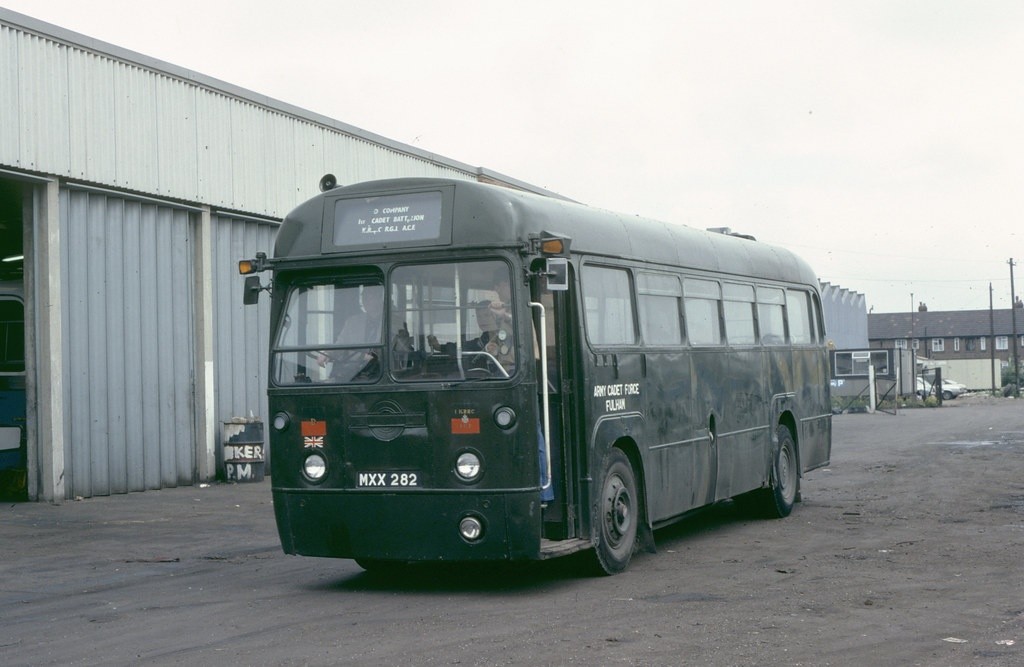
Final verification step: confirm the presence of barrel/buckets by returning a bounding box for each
[223,421,266,483]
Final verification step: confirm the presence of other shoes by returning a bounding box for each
[541,501,548,508]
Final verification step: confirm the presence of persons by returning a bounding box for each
[317,286,382,381]
[427,301,496,369]
[485,264,555,501]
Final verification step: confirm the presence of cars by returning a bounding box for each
[916,378,968,400]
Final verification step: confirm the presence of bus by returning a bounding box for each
[0,279,25,499]
[239,176,832,577]
